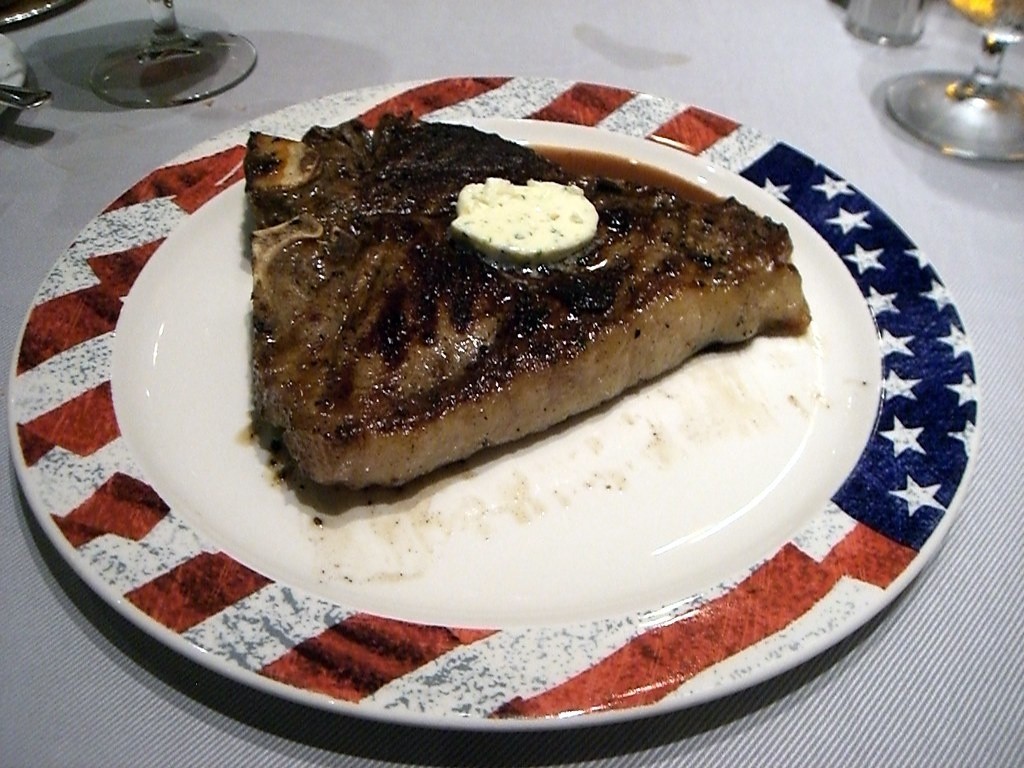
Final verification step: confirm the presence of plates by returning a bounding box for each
[0,33,28,117]
[6,78,984,731]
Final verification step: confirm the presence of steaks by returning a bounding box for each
[239,110,812,489]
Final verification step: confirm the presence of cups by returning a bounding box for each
[843,0,928,46]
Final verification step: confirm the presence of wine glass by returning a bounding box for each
[87,1,258,109]
[886,0,1023,161]
